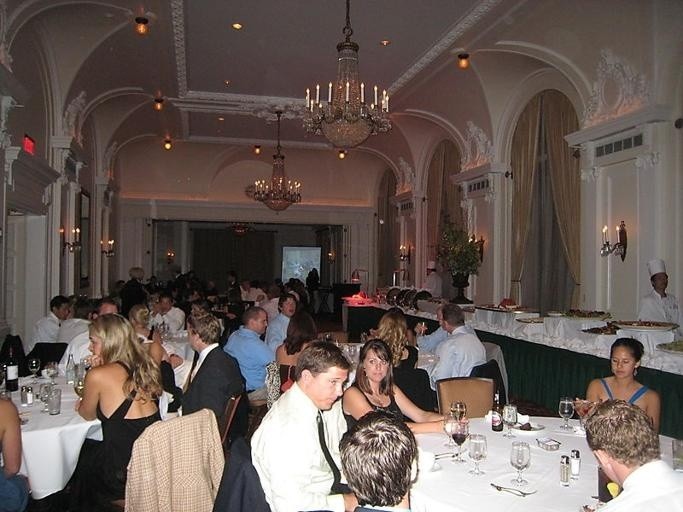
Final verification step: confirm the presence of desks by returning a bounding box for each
[347,301,683,441]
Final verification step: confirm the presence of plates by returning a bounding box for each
[464,302,540,314]
[561,313,612,321]
[610,320,679,331]
[657,342,683,356]
[386,287,433,311]
[547,310,564,317]
[581,328,618,336]
[513,318,543,324]
[516,422,546,432]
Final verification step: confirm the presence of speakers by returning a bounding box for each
[333,284,360,321]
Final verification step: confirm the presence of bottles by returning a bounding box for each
[5,343,19,392]
[65,353,76,386]
[560,450,581,487]
[21,385,33,407]
[491,388,504,431]
[78,359,90,379]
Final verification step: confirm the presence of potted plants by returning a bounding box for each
[433,222,483,304]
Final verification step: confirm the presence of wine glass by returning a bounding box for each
[510,439,531,487]
[558,396,575,432]
[501,404,517,441]
[47,361,59,386]
[38,382,53,414]
[322,332,358,366]
[468,434,488,476]
[443,400,469,464]
[27,358,41,386]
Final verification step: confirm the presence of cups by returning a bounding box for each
[156,326,192,343]
[670,439,683,472]
[48,389,61,415]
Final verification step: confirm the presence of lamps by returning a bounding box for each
[350,268,369,282]
[304,0,393,160]
[458,52,470,69]
[60,223,83,257]
[135,17,172,151]
[255,145,261,154]
[399,244,411,264]
[99,237,115,257]
[600,219,627,264]
[226,221,257,237]
[251,110,302,218]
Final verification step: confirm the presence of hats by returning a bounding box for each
[427,261,435,269]
[648,259,666,277]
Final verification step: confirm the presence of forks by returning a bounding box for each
[492,484,540,503]
[435,451,452,460]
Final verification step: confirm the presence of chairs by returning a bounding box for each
[121,357,280,512]
[387,339,511,420]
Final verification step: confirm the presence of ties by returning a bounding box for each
[317,417,341,489]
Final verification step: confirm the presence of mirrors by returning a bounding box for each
[80,185,91,288]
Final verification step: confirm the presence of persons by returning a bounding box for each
[35,296,246,495]
[579,397,683,510]
[377,302,487,407]
[116,265,316,406]
[250,339,468,511]
[0,397,30,511]
[574,337,661,433]
[637,273,679,323]
